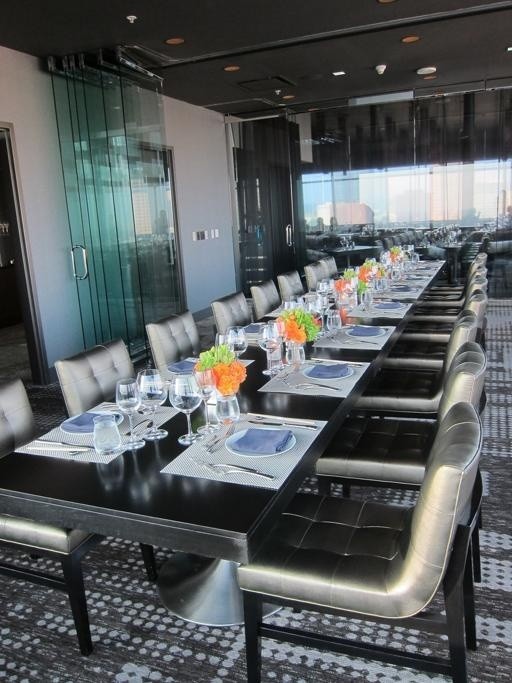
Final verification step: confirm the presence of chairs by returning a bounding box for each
[415,230,423,240]
[461,232,489,276]
[392,236,402,246]
[350,309,482,418]
[236,402,483,683]
[211,291,252,334]
[250,279,281,319]
[145,310,201,369]
[277,270,304,302]
[315,341,487,583]
[428,264,488,294]
[400,233,409,244]
[304,262,328,290]
[399,274,488,349]
[374,239,385,260]
[54,336,137,418]
[383,237,393,250]
[418,254,486,310]
[406,231,416,242]
[389,289,488,366]
[411,267,488,326]
[0,375,157,656]
[317,256,337,277]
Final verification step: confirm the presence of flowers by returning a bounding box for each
[390,246,401,262]
[276,306,321,344]
[194,345,247,395]
[357,261,386,283]
[335,268,367,295]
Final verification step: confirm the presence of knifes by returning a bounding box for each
[248,420,319,430]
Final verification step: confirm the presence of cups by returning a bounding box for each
[214,331,234,353]
[215,392,241,426]
[360,286,372,312]
[93,414,122,454]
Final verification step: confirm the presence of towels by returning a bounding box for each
[170,360,198,373]
[309,364,349,379]
[392,286,411,292]
[351,326,380,337]
[377,302,400,309]
[244,324,262,332]
[62,411,119,433]
[407,276,422,280]
[418,266,431,270]
[232,428,292,453]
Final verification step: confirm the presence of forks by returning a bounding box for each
[206,425,236,453]
[191,457,259,473]
[203,463,276,480]
[280,379,339,391]
[291,386,341,390]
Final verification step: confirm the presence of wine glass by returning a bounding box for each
[194,370,220,434]
[286,342,304,372]
[135,369,168,442]
[327,310,341,342]
[169,372,204,445]
[225,326,248,359]
[268,320,288,369]
[116,378,146,450]
[258,324,282,376]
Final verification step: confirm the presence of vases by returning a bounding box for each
[215,396,240,424]
[286,341,305,364]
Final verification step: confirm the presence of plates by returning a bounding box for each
[303,366,354,381]
[60,410,125,436]
[225,427,297,458]
[373,304,406,312]
[345,329,386,338]
